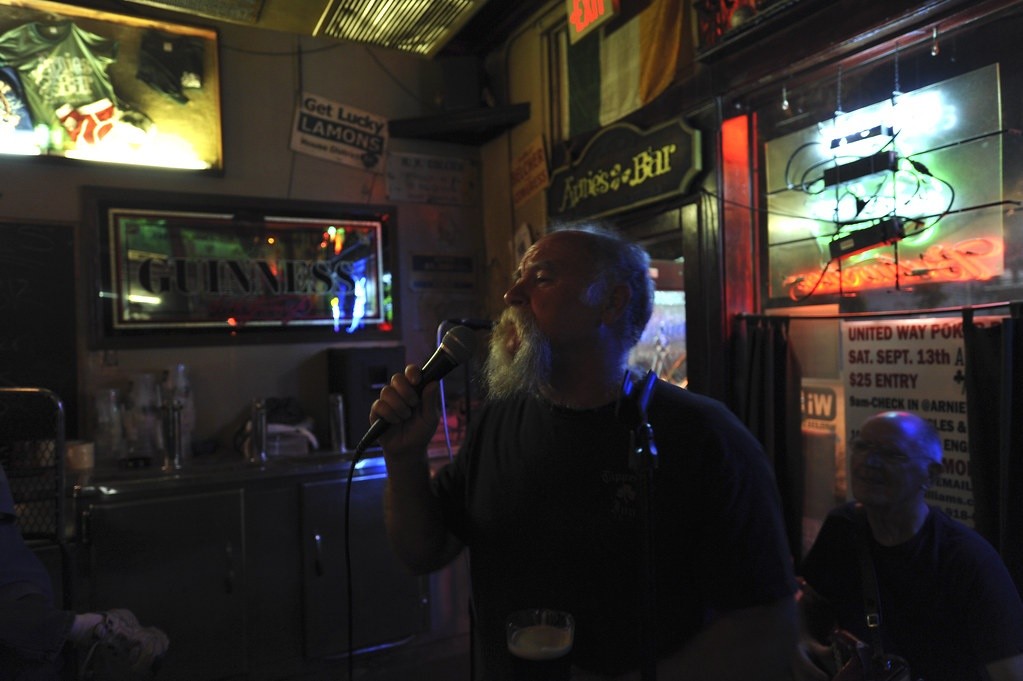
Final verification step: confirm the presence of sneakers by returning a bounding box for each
[83,607,169,677]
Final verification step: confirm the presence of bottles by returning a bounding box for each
[96,362,195,462]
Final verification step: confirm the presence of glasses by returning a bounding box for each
[848,437,939,467]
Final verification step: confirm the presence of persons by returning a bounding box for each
[791,410,1023,681]
[371,229,797,681]
[0,466,170,681]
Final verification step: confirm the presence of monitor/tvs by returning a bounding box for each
[759,61,1009,311]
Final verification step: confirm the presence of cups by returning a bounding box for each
[504,609,575,681]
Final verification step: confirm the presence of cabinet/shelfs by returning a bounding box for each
[81,450,482,681]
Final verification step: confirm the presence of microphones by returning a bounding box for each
[351,324,479,460]
